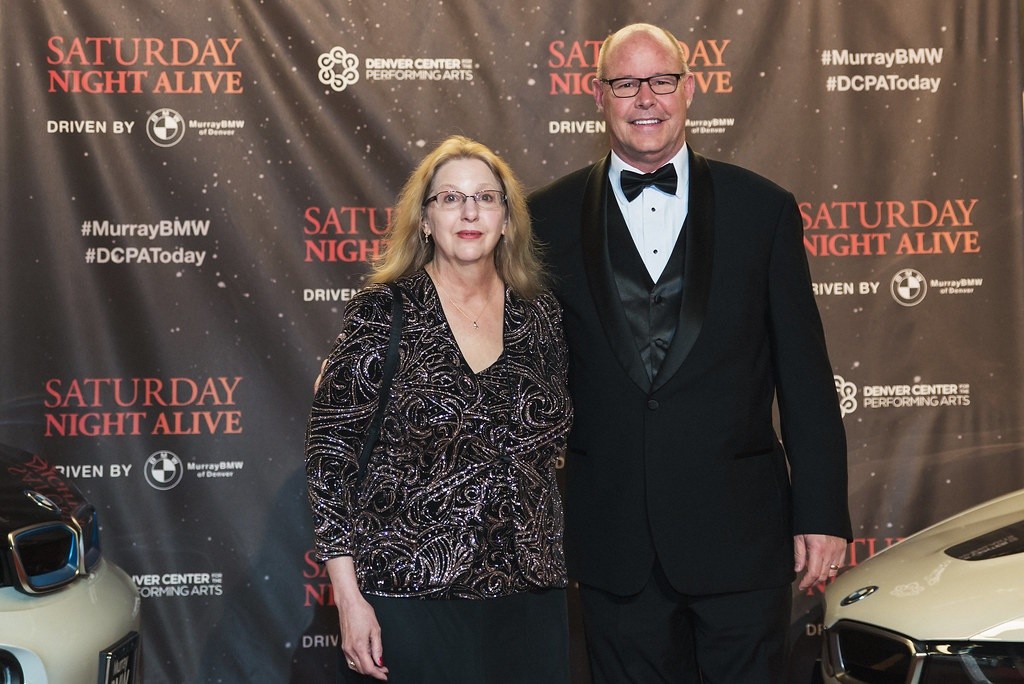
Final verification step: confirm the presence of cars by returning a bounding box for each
[789,486,1024,684]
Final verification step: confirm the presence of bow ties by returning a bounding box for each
[620,163,679,202]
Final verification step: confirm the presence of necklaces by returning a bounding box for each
[424,265,499,331]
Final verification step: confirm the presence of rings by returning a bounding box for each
[830,564,838,570]
[350,661,356,668]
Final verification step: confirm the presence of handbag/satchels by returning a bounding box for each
[289,604,358,684]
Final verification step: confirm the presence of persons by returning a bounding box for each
[524,23,854,684]
[304,136,576,683]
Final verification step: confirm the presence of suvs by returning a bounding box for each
[0,443,142,684]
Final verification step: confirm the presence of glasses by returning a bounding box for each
[422,190,508,209]
[598,70,687,98]
